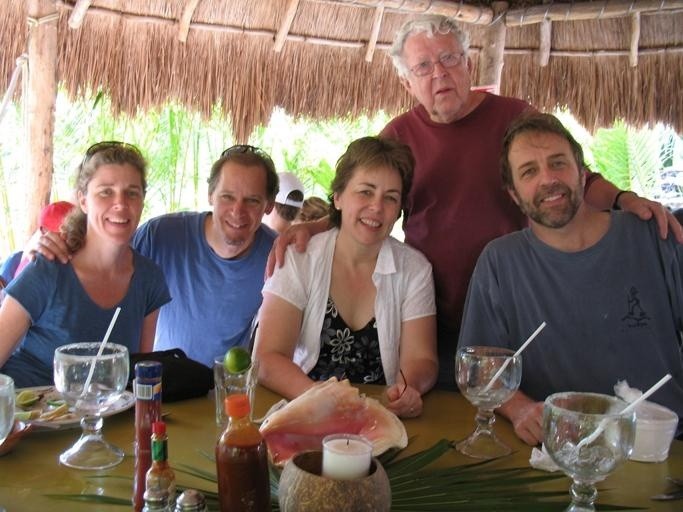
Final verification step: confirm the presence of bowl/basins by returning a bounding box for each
[628,403,678,464]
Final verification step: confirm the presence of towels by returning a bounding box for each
[529,441,561,471]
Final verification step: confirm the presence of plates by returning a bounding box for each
[13,383,136,431]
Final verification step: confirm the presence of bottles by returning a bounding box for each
[215,394,270,512]
[143,422,175,510]
[175,492,206,512]
[132,362,162,510]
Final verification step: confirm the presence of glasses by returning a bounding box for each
[403,52,464,76]
[82,140,140,166]
[341,368,408,404]
[221,145,273,163]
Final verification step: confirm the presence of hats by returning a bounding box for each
[274,172,305,208]
[40,202,74,234]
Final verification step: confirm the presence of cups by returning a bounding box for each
[214,356,256,430]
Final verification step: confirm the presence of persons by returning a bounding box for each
[262,12,683,393]
[249,137,440,422]
[263,171,305,235]
[0,200,79,288]
[454,113,683,448]
[0,140,173,390]
[20,145,283,370]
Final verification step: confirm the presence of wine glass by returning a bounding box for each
[454,346,522,458]
[543,392,638,512]
[0,372,15,446]
[54,342,129,470]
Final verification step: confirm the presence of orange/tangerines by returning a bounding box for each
[223,346,252,375]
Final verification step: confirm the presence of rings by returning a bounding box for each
[411,407,414,414]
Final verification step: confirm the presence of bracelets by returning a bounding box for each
[611,190,629,211]
